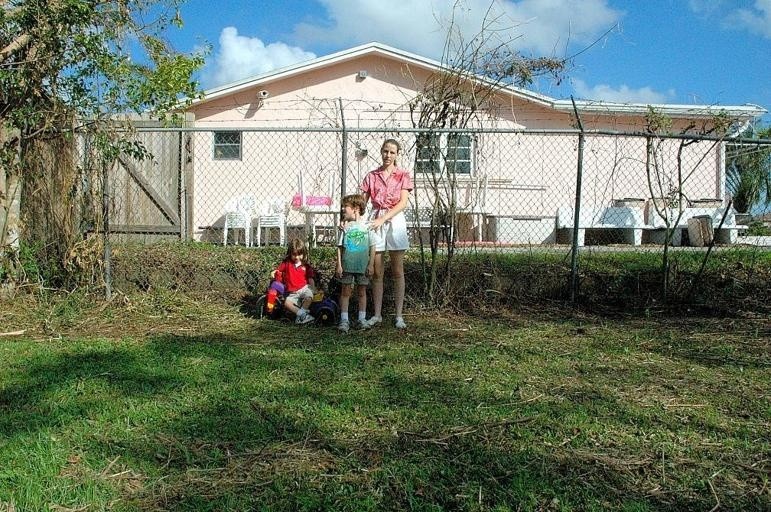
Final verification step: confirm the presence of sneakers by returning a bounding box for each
[340,321,350,332]
[395,318,407,328]
[295,313,313,324]
[359,316,382,331]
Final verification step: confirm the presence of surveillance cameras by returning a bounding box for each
[257,90,269,99]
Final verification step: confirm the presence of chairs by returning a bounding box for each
[222,195,292,248]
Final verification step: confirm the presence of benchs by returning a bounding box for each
[404,208,451,245]
[556,207,748,248]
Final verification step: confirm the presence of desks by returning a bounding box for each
[456,209,493,242]
[297,169,342,250]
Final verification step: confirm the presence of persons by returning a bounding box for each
[334,194,377,332]
[268,238,319,325]
[338,138,414,329]
